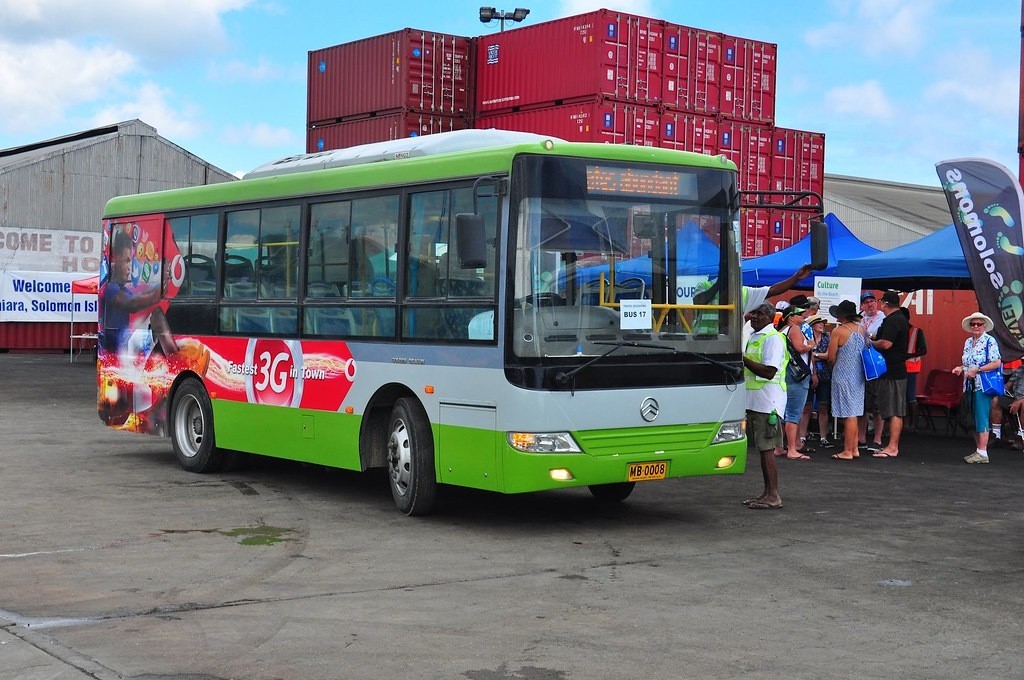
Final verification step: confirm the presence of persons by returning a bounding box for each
[693,255,927,509]
[439,239,492,340]
[950,311,1024,464]
[102,230,209,382]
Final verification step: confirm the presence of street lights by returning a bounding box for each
[479,6,529,31]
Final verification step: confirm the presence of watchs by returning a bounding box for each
[815,353,819,358]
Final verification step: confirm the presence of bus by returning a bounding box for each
[94,128,829,518]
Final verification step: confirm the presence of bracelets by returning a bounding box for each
[808,345,811,349]
[978,367,982,373]
[792,273,799,282]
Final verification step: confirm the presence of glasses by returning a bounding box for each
[789,312,803,316]
[970,322,986,327]
[800,304,810,310]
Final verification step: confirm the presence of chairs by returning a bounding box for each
[914,368,966,440]
[1000,398,1022,445]
[182,250,645,340]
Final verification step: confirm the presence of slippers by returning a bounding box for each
[872,450,898,458]
[742,494,784,509]
[774,446,817,461]
[831,451,861,460]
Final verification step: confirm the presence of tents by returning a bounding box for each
[557,214,975,290]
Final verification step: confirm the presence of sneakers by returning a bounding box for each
[857,440,869,448]
[799,437,807,445]
[819,439,834,448]
[867,442,884,450]
[964,452,990,463]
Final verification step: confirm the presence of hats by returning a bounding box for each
[790,295,817,310]
[806,315,827,326]
[775,300,790,311]
[784,306,807,319]
[829,300,857,318]
[861,293,875,303]
[878,291,900,305]
[962,312,995,333]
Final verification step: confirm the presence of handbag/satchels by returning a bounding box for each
[906,357,922,373]
[861,340,887,382]
[979,337,1004,396]
[957,376,975,429]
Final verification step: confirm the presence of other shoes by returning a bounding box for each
[1012,435,1024,451]
[986,433,1002,448]
